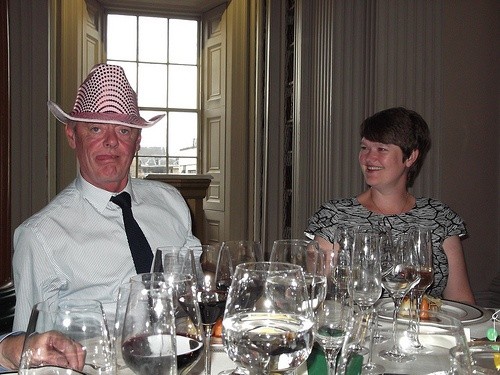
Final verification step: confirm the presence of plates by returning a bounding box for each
[377,295,492,326]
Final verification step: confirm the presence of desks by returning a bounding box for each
[79,299,500,375]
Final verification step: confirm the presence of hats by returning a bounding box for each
[45,63,165,129]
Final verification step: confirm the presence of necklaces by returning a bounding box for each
[371,192,408,215]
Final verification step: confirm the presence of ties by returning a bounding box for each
[109,192,164,289]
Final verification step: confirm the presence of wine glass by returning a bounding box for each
[21,223,476,375]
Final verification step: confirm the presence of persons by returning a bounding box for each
[12,64,204,342]
[0,330,86,373]
[303,107,476,307]
